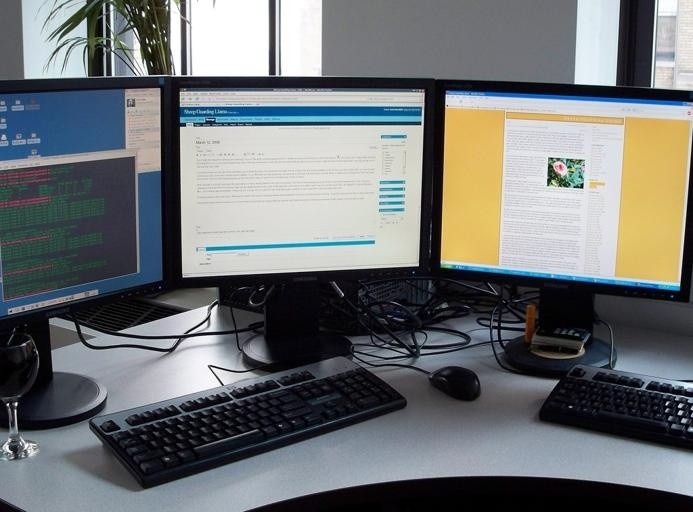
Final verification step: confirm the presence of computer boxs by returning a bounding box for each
[218,275,443,336]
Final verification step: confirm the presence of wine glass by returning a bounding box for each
[0,335,40,460]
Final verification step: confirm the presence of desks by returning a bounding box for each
[0,300,693,512]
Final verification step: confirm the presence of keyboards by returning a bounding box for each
[537,363,693,456]
[89,356,407,490]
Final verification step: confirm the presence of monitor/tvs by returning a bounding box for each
[169,75,435,372]
[0,76,169,432]
[432,78,693,377]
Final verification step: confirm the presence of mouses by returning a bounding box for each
[429,365,481,401]
